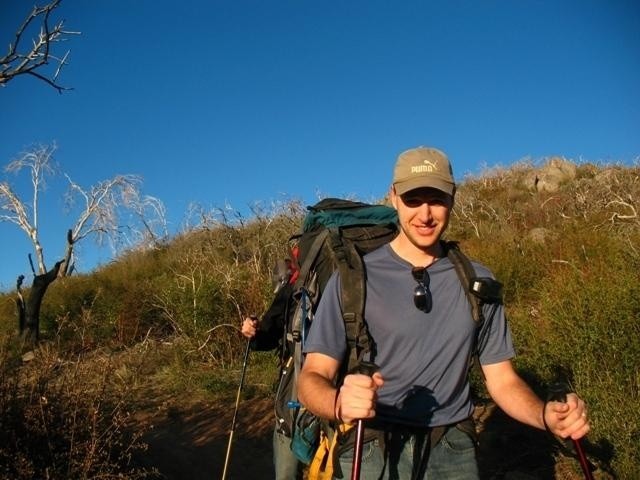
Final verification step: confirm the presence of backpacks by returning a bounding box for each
[297,198,483,348]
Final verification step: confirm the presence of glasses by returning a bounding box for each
[412,267,431,313]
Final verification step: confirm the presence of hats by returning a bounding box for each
[393,146,455,196]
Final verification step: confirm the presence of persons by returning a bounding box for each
[289,144,598,480]
[238,277,321,480]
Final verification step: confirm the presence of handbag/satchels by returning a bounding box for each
[290,408,320,465]
